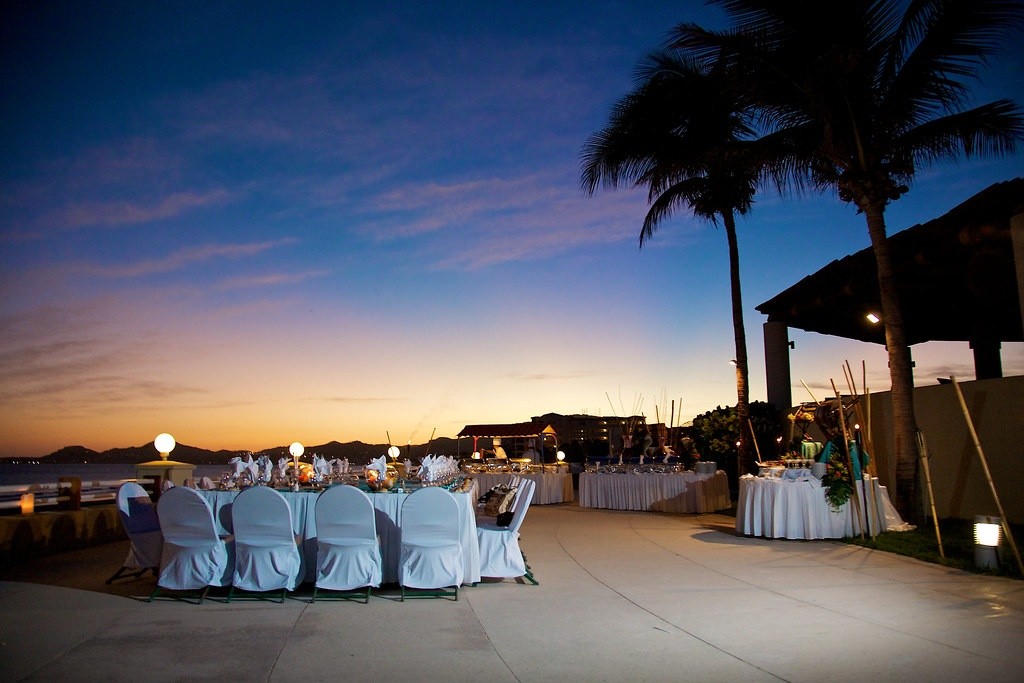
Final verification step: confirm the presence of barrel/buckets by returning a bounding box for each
[695,461,716,473]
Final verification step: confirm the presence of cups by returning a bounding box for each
[421,468,454,487]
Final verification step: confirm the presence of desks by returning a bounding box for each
[0,459,880,562]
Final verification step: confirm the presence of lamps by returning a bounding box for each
[289,442,305,459]
[557,451,566,461]
[154,433,176,461]
[388,446,401,460]
[20,492,35,517]
[972,515,1002,568]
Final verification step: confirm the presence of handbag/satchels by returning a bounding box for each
[477,483,522,518]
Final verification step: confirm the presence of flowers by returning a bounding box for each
[821,433,854,507]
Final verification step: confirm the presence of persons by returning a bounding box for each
[522,438,540,465]
[481,438,507,458]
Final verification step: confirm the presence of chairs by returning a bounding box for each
[104,474,540,605]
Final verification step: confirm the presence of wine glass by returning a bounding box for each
[222,471,230,492]
[374,475,385,493]
[257,472,264,486]
[240,471,248,490]
[785,458,814,469]
